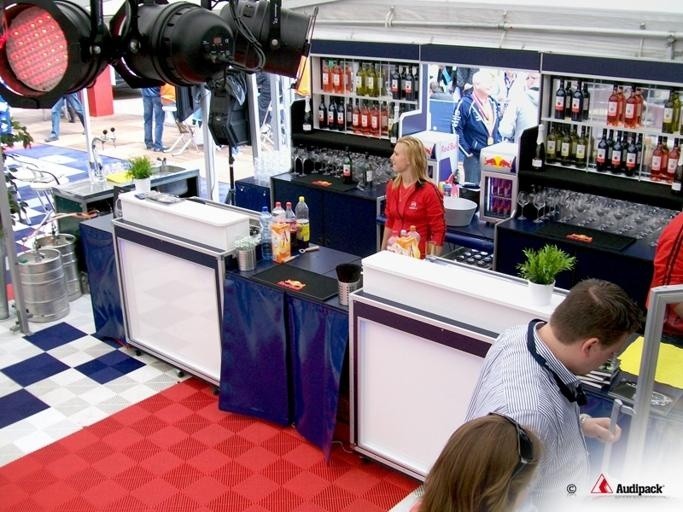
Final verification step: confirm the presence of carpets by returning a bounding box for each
[1,377,422,512]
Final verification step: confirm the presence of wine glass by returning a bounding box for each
[489,176,512,219]
[291,145,398,193]
[528,184,680,247]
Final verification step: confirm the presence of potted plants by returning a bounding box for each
[125,154,154,198]
[510,244,578,309]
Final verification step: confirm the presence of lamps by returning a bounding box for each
[0,0,314,110]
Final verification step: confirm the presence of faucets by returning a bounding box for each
[445,168,461,199]
[91,126,119,181]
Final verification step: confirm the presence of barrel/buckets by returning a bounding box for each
[31,233,84,302]
[16,248,70,324]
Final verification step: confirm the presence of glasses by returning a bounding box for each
[488,412,532,483]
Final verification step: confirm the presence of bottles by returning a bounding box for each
[301,94,312,131]
[258,196,313,268]
[316,59,421,146]
[456,160,464,187]
[438,179,452,196]
[86,147,106,186]
[546,78,682,183]
[531,123,545,172]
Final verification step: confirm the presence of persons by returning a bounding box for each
[430,63,542,190]
[459,277,641,511]
[42,88,85,144]
[139,84,170,153]
[385,414,545,512]
[256,71,285,128]
[377,133,447,262]
[642,211,682,351]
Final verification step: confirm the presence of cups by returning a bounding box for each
[236,247,256,272]
[334,263,362,307]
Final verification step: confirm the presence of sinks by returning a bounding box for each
[128,164,189,195]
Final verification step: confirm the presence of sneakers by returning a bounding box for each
[45,136,59,142]
[154,145,168,152]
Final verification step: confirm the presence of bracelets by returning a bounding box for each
[577,411,592,426]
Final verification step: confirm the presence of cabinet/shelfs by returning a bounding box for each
[404,131,458,190]
[490,216,657,332]
[538,71,683,187]
[234,180,271,213]
[309,54,420,141]
[271,171,399,255]
